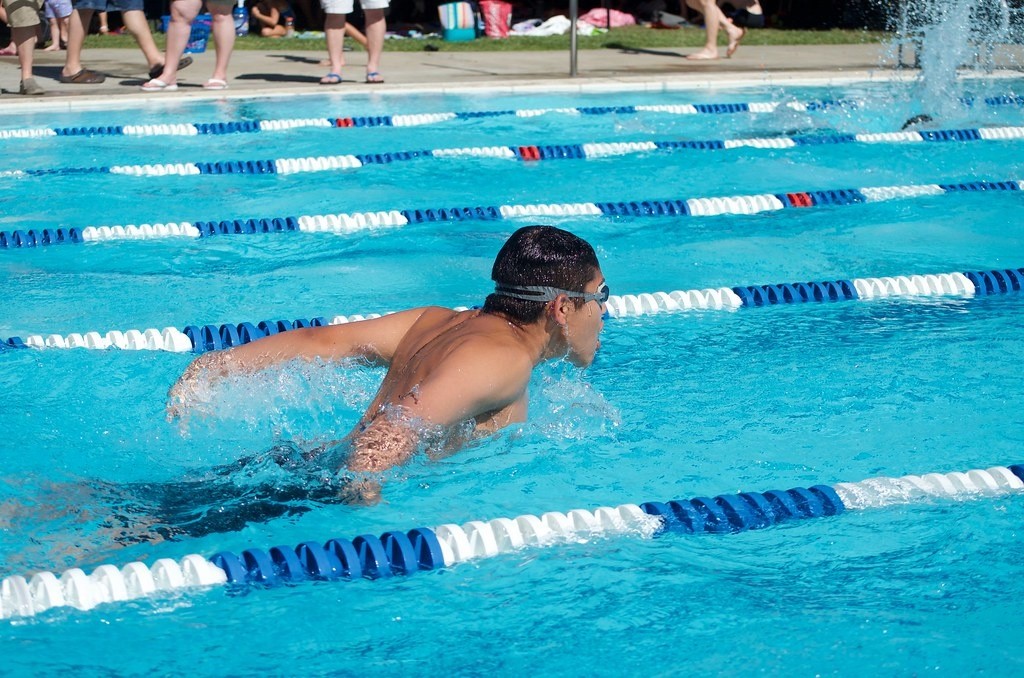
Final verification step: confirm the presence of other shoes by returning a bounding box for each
[20,78,45,95]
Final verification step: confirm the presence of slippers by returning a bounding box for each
[204,79,228,89]
[61,69,107,84]
[140,79,178,90]
[320,74,341,83]
[367,72,385,83]
[686,51,720,61]
[149,56,192,77]
[727,28,748,58]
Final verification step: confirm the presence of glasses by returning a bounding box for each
[495,286,611,303]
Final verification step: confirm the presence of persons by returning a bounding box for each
[54,0,193,84]
[684,0,747,61]
[44,0,74,51]
[318,20,368,67]
[248,0,286,38]
[0,0,47,96]
[140,0,237,90]
[95,9,110,36]
[2,225,609,581]
[318,0,389,85]
